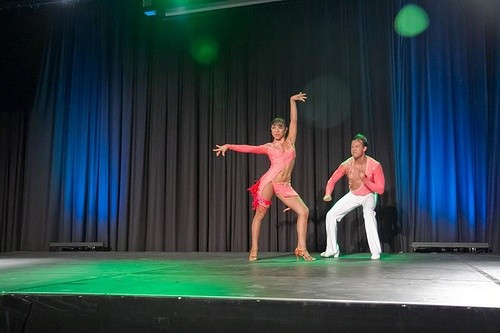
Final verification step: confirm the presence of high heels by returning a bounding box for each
[249,249,258,261]
[321,250,340,258]
[295,248,316,261]
[370,253,381,260]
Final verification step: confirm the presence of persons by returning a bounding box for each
[321,134,385,261]
[213,91,315,262]
